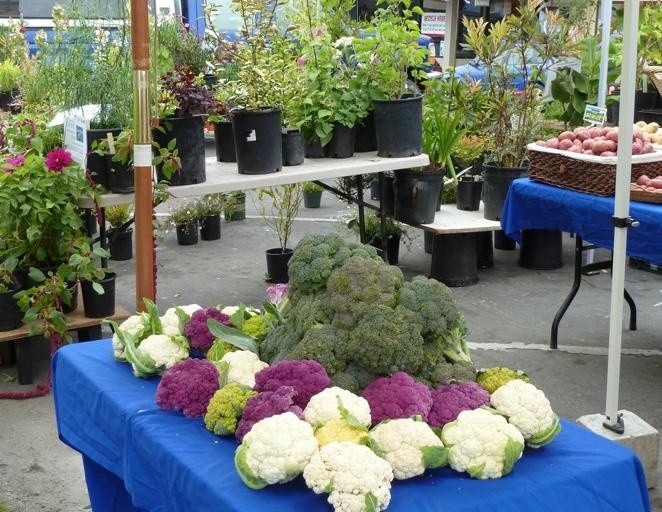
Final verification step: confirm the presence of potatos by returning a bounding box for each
[534,118,662,192]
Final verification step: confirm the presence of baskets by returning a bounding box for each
[526,151,662,198]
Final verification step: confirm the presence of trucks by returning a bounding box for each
[205,1,445,94]
[0,0,209,111]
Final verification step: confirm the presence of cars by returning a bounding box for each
[429,41,618,122]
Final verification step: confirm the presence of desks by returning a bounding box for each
[499,178,662,348]
[52,338,653,512]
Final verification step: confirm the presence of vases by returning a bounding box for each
[476,231,494,267]
[84,270,116,318]
[455,174,483,210]
[429,232,478,287]
[516,227,566,268]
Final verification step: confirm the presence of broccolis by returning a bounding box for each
[110,232,562,512]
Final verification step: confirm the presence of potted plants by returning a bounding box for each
[80,26,133,190]
[105,204,133,260]
[224,190,246,222]
[304,182,325,207]
[197,193,220,242]
[172,203,197,246]
[203,1,367,175]
[1,1,84,387]
[368,1,423,157]
[151,67,209,186]
[371,110,465,226]
[463,1,590,249]
